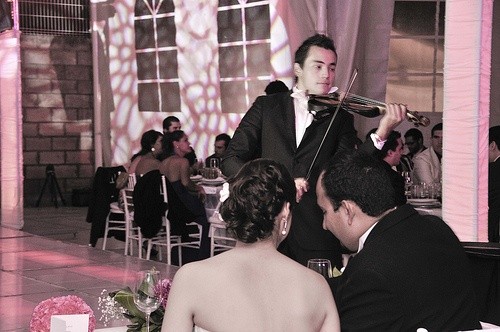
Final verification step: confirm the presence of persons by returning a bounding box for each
[315,151,484,332]
[485,126,500,243]
[263,80,289,96]
[161,158,341,332]
[222,32,407,270]
[365,119,443,195]
[128,115,231,257]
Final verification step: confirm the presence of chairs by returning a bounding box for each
[97,166,145,258]
[139,173,202,266]
[208,197,237,258]
[117,171,164,261]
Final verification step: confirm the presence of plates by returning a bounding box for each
[189,176,225,184]
[406,198,438,203]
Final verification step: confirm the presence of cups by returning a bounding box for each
[210,158,219,169]
[410,182,441,199]
[307,258,332,279]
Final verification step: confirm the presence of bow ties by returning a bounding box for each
[290,90,308,107]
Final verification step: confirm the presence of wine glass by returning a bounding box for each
[133,270,164,332]
[195,158,202,175]
[402,171,413,199]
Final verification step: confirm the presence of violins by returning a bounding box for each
[308,92,431,128]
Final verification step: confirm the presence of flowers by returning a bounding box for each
[29,294,97,332]
[332,266,346,278]
[98,265,173,332]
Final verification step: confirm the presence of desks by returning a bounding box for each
[189,174,226,195]
